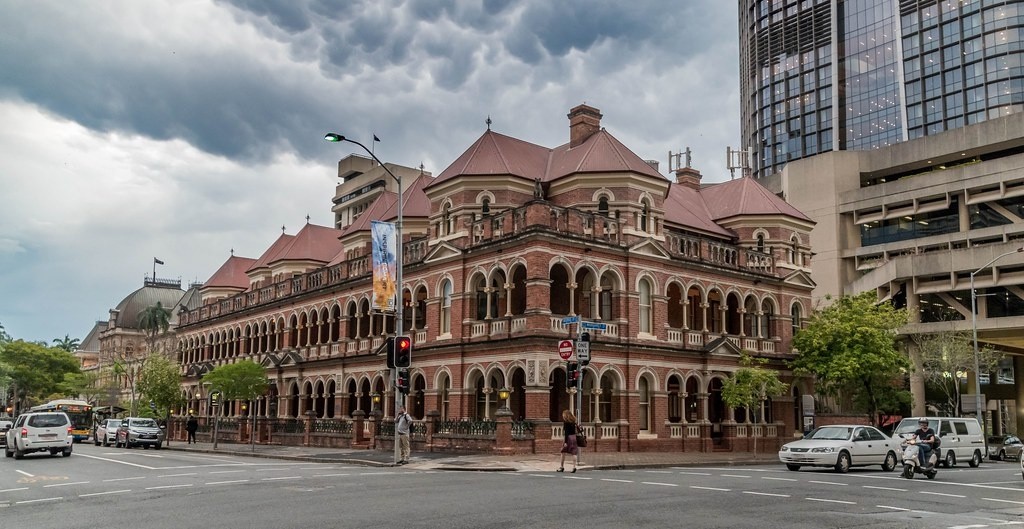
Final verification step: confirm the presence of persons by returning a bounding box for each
[394,406,413,465]
[556,410,578,474]
[897,417,935,478]
[185,416,199,444]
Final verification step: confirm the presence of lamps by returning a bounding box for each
[240,402,247,415]
[372,390,380,411]
[498,385,511,410]
[169,408,174,414]
[189,407,194,415]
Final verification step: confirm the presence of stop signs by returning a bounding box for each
[559,341,573,359]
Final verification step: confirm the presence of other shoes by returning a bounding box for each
[921,466,926,470]
[402,461,408,464]
[397,459,404,464]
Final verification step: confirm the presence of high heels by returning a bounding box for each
[557,468,564,472]
[570,468,576,473]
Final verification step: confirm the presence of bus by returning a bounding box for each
[28,399,94,442]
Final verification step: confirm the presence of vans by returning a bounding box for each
[890,416,987,468]
[4,412,73,460]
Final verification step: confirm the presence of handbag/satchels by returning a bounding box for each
[576,435,587,447]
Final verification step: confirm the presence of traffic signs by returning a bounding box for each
[577,341,590,360]
[561,316,579,325]
[582,321,607,331]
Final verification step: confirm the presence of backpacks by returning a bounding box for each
[398,413,416,433]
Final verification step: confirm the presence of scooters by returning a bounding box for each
[898,434,941,481]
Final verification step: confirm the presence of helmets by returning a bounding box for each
[918,417,929,423]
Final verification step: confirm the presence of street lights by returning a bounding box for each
[970,245,1024,428]
[324,130,406,467]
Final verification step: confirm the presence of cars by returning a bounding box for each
[114,417,166,449]
[94,418,124,447]
[777,424,903,473]
[985,434,1024,460]
[0,417,14,446]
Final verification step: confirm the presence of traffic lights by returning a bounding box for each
[565,362,578,388]
[397,369,410,394]
[394,335,412,368]
